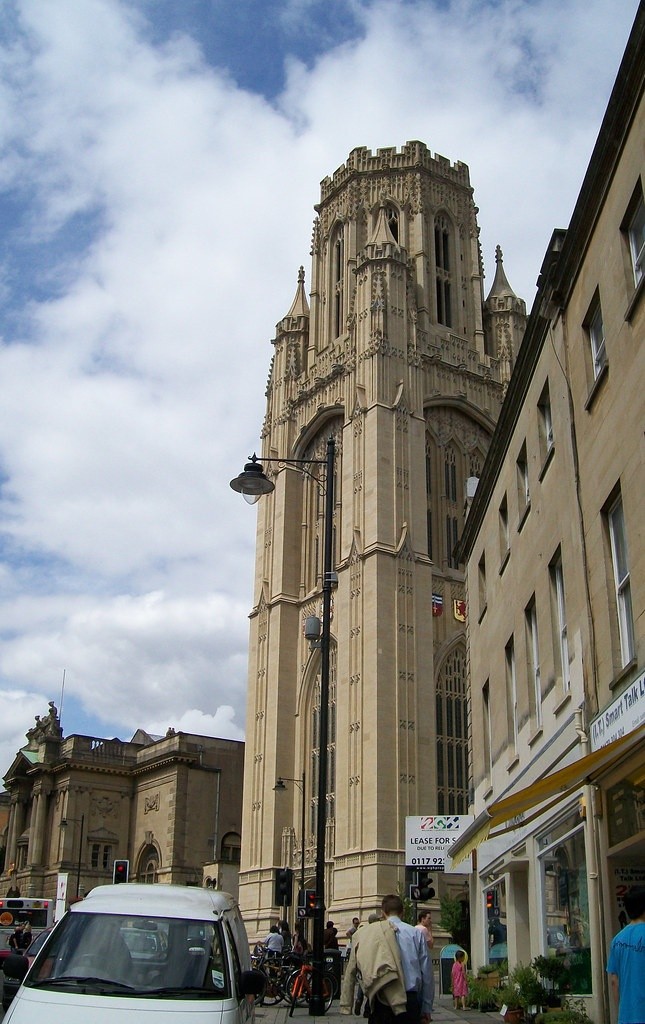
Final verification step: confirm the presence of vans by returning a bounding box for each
[1,883,266,1024]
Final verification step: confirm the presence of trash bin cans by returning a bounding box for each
[324,949,342,1000]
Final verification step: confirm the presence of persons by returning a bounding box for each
[11,924,32,954]
[152,938,254,1010]
[48,701,57,733]
[607,887,645,1024]
[8,864,18,876]
[414,912,434,949]
[98,933,135,984]
[452,951,471,1010]
[324,920,340,949]
[35,716,42,726]
[346,918,359,958]
[355,914,380,1017]
[367,895,435,1024]
[253,920,307,982]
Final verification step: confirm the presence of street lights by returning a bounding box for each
[58,817,84,898]
[272,777,306,906]
[231,453,335,1017]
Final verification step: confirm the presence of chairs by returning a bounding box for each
[79,931,136,985]
[157,938,214,989]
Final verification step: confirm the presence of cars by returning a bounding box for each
[2,926,55,1013]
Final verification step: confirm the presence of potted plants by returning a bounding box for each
[450,955,595,1024]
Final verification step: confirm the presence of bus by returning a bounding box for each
[0,897,54,959]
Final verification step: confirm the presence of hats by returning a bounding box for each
[369,913,383,923]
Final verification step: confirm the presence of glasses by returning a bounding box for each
[353,922,360,926]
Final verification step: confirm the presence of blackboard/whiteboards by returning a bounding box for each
[439,958,467,995]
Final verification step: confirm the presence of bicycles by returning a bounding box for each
[251,941,339,1017]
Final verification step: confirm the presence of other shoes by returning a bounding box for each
[363,1012,371,1018]
[355,1008,359,1014]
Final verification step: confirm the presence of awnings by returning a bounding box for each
[447,723,645,869]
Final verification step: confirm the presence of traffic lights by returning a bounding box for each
[487,889,495,917]
[113,860,129,883]
[305,889,316,917]
[420,877,435,900]
